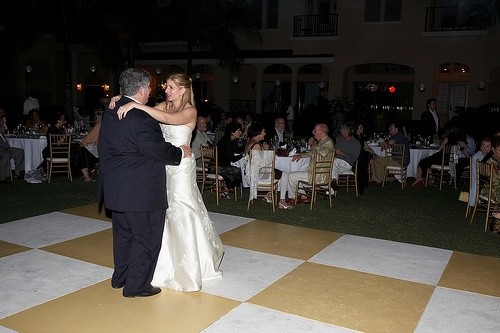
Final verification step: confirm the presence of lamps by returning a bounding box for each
[88,64,97,75]
[232,75,240,84]
[23,64,32,74]
[418,82,426,92]
[154,67,162,77]
[366,80,398,93]
[477,80,486,90]
[319,82,326,91]
[274,80,282,88]
[195,72,202,80]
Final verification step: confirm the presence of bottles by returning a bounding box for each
[379,132,386,144]
[75,124,86,134]
[373,132,376,142]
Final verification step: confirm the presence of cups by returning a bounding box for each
[288,140,292,146]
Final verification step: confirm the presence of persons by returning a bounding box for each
[109,74,225,291]
[0,88,500,233]
[98,68,192,298]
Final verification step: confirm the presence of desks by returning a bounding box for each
[370,144,392,157]
[6,138,48,174]
[394,149,438,183]
[275,157,310,191]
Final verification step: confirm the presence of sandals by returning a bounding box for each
[287,199,299,206]
[298,197,309,204]
[221,193,231,200]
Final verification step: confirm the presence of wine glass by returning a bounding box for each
[270,135,279,150]
[297,136,302,146]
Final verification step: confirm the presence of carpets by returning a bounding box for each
[0,203,500,333]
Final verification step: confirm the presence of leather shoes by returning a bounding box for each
[111,281,126,288]
[123,286,161,298]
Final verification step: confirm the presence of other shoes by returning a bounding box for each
[279,203,294,209]
[263,196,272,203]
[325,195,335,200]
[411,179,424,187]
[0,179,12,184]
[85,178,96,183]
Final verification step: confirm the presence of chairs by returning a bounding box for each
[46,131,500,231]
